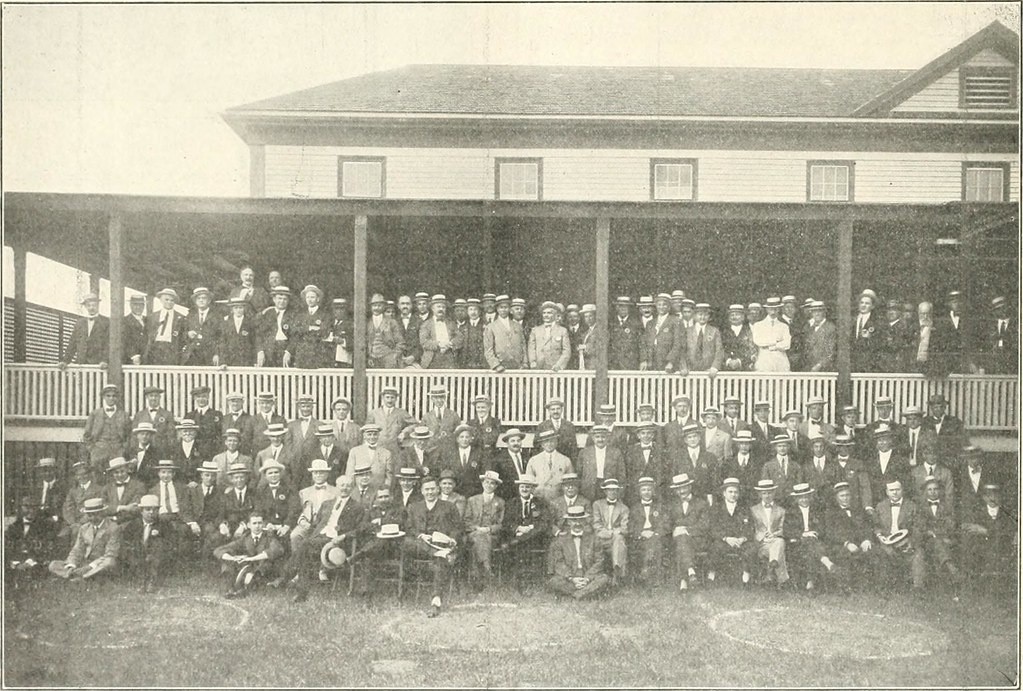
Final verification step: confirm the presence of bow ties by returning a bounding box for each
[24,522,31,526]
[928,501,938,506]
[116,482,126,486]
[642,502,650,506]
[891,503,900,506]
[607,501,616,505]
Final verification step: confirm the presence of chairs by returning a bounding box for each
[304,535,953,600]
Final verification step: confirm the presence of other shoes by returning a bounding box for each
[427,604,440,615]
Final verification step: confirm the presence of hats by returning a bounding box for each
[138,495,162,506]
[886,528,916,550]
[34,384,985,496]
[376,524,407,539]
[18,496,41,503]
[80,497,109,512]
[196,461,220,472]
[77,284,1006,309]
[422,530,453,553]
[235,565,255,593]
[563,506,590,518]
[980,480,1002,496]
[321,543,347,570]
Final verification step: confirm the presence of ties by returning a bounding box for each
[164,483,172,513]
[206,488,209,494]
[239,492,243,502]
[523,501,526,516]
[569,500,572,505]
[336,500,340,512]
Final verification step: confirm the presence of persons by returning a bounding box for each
[117,383,324,469]
[211,511,284,599]
[365,290,595,423]
[82,384,133,474]
[609,288,837,421]
[118,494,175,587]
[184,422,348,578]
[464,396,631,595]
[620,393,1016,599]
[49,498,121,582]
[4,496,53,596]
[343,385,487,521]
[845,284,1020,430]
[26,419,207,567]
[269,474,372,603]
[52,264,357,415]
[544,505,612,600]
[405,473,466,616]
